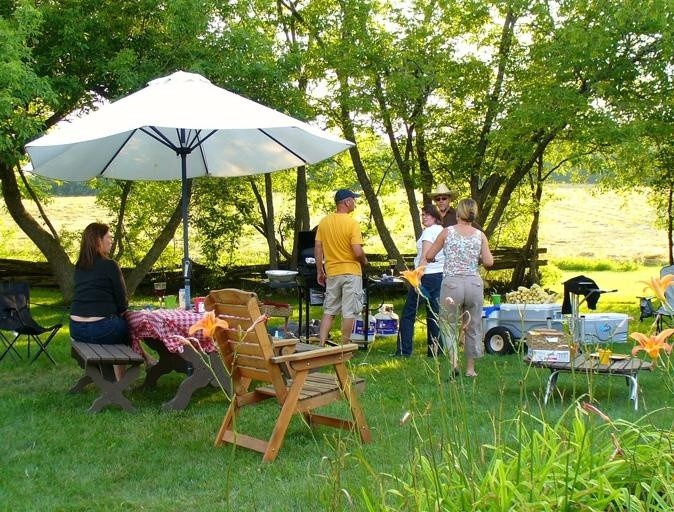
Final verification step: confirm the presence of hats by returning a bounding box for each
[334,189,363,202]
[425,183,459,202]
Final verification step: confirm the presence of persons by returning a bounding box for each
[385,201,446,361]
[424,198,495,384]
[425,183,460,227]
[312,186,369,348]
[65,221,158,394]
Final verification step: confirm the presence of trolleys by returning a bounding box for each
[482,281,620,356]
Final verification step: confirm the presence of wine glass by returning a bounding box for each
[388,258,398,278]
[153,281,168,311]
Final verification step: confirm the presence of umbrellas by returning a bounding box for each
[24,70,358,314]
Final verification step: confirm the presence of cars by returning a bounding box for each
[297,225,325,281]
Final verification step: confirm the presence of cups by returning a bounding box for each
[597,350,611,365]
[161,286,207,313]
[491,294,501,306]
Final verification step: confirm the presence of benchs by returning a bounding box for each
[68,341,144,414]
[279,344,320,373]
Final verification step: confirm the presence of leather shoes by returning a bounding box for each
[443,367,461,383]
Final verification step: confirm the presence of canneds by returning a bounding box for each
[179,289,185,308]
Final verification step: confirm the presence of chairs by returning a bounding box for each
[0,282,63,367]
[204,288,372,464]
[655,265,674,335]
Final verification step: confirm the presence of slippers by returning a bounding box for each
[464,369,478,377]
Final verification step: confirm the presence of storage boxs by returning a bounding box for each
[500,304,563,339]
[526,331,571,350]
[578,314,628,343]
[528,349,570,362]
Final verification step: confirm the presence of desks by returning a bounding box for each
[524,351,650,410]
[240,276,404,350]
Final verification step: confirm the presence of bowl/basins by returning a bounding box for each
[266,267,300,283]
